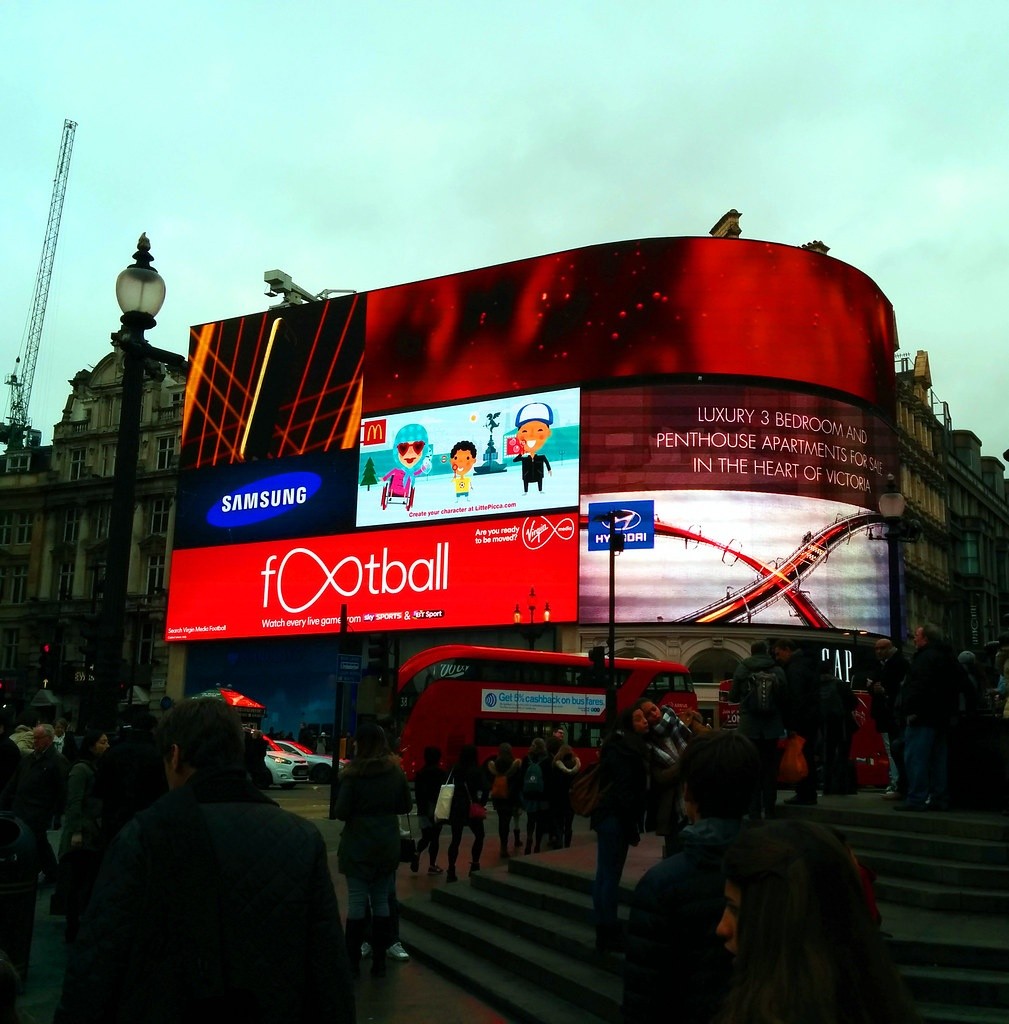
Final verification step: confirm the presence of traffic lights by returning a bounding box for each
[38,642,55,680]
[588,646,605,686]
[78,611,102,665]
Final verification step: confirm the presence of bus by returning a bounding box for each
[718,678,891,790]
[384,644,698,780]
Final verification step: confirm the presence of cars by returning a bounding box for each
[243,726,311,791]
[272,739,352,784]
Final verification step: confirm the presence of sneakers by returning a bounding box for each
[410,865,419,875]
[387,942,410,960]
[361,942,371,958]
[427,864,443,875]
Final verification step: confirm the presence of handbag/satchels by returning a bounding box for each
[402,839,416,862]
[776,732,809,783]
[433,784,455,820]
[469,803,487,820]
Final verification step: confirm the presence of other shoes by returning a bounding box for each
[446,867,457,883]
[535,847,540,853]
[501,850,512,859]
[880,791,910,801]
[526,850,530,855]
[371,959,387,980]
[468,862,480,877]
[783,793,818,806]
[894,802,928,812]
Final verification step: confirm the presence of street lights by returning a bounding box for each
[591,510,633,739]
[82,228,168,738]
[878,473,907,699]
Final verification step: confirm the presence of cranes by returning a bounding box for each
[0,117,80,453]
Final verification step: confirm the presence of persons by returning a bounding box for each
[0,713,170,1024]
[716,819,922,1024]
[818,657,860,794]
[445,746,489,881]
[774,638,820,805]
[334,722,412,967]
[729,640,790,822]
[266,727,294,741]
[590,698,694,952]
[299,723,328,754]
[55,698,359,1024]
[873,625,1009,812]
[411,747,445,873]
[620,729,779,1024]
[488,729,581,857]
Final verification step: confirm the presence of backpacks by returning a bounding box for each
[570,763,614,816]
[525,755,550,793]
[741,667,779,717]
[491,776,508,797]
